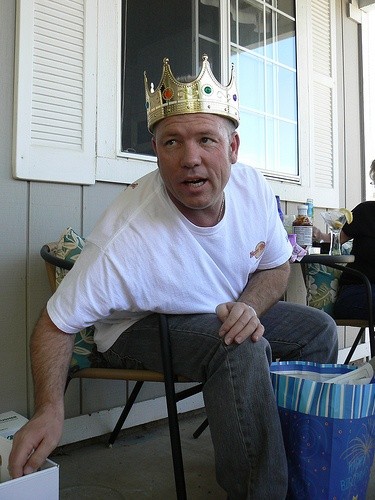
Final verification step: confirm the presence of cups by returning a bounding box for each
[282,215,296,234]
[287,234,297,246]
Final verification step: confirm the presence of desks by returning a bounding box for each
[277,255,355,302]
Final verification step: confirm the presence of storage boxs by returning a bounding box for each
[0,458,60,500]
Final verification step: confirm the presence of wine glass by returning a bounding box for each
[320,210,353,256]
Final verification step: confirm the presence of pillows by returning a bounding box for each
[305,240,353,317]
[53,228,94,373]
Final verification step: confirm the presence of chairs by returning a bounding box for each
[300,261,375,366]
[40,241,205,500]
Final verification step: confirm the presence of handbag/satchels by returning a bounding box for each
[269,361,375,500]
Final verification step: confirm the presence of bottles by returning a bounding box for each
[275,196,283,222]
[305,198,313,219]
[292,204,313,255]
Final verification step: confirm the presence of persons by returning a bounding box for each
[8,73,340,500]
[310,161,375,322]
